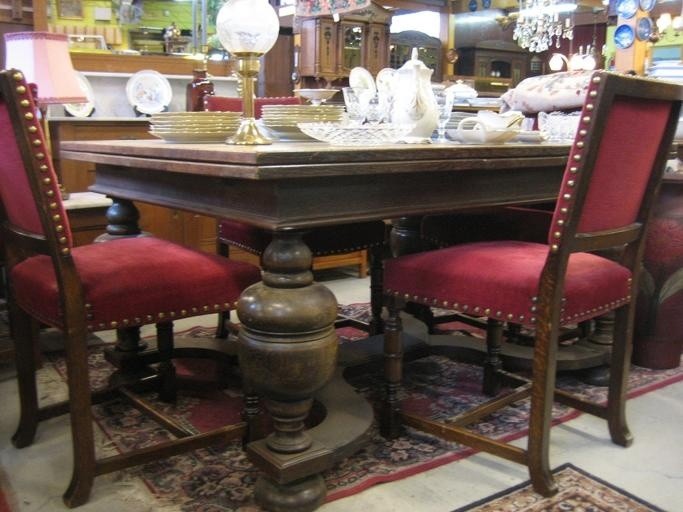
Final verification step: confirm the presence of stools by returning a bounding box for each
[61,194,111,230]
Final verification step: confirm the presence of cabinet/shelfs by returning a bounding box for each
[299,1,391,79]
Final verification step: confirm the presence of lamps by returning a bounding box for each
[549,42,597,73]
[513,0,578,52]
[215,1,280,146]
[4,31,90,199]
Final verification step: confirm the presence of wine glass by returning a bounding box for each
[434,90,456,142]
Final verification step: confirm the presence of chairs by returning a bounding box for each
[379,68,683,499]
[203,90,386,340]
[0,68,261,509]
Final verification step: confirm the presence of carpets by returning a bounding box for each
[42,302,682,511]
[451,462,664,511]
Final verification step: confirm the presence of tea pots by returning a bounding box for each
[392,45,438,144]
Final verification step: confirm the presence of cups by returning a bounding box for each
[345,85,391,122]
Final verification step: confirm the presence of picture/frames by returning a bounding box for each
[33,0,245,79]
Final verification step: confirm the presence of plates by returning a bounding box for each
[126,69,172,115]
[639,1,655,13]
[539,110,582,141]
[613,24,633,49]
[448,118,521,143]
[259,105,346,139]
[346,68,375,96]
[293,88,341,101]
[636,17,654,41]
[61,71,96,118]
[375,67,397,88]
[298,121,401,145]
[614,0,638,19]
[148,111,244,143]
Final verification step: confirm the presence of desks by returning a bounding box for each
[58,129,683,436]
